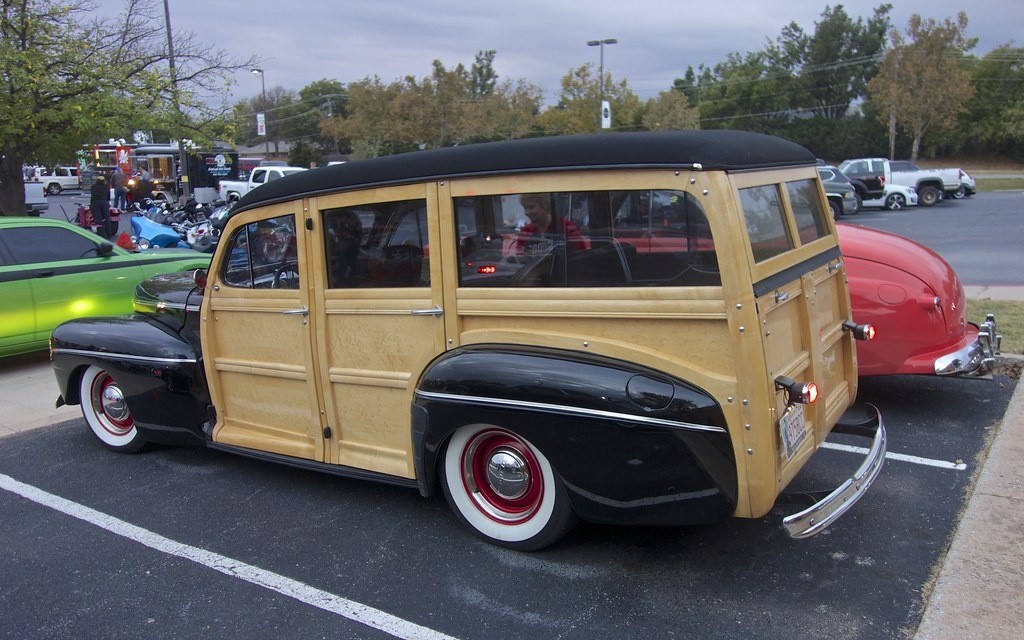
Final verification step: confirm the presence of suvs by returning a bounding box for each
[834,165,883,210]
[816,165,858,221]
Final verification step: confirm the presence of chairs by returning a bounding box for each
[460,233,504,262]
[372,244,427,287]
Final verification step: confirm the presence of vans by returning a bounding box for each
[34,167,79,195]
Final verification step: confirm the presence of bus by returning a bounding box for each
[80,143,240,205]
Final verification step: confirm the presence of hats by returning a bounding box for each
[114,167,121,172]
[97,176,105,181]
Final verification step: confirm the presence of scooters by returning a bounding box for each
[75,199,120,234]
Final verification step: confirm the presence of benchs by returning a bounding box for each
[544,243,637,285]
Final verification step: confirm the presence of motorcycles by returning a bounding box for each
[130,197,236,254]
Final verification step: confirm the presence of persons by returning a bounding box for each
[517,192,587,257]
[110,167,128,214]
[120,185,153,216]
[139,164,150,181]
[131,169,141,178]
[90,175,113,238]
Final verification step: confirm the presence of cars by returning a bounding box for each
[888,161,919,171]
[47,130,887,553]
[0,217,215,358]
[953,168,976,199]
[862,184,917,211]
[498,212,1004,383]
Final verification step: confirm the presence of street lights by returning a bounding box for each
[252,69,269,159]
[587,39,618,132]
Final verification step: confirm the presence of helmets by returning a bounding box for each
[131,171,141,177]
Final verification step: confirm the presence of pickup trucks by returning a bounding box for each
[218,167,308,205]
[834,159,961,207]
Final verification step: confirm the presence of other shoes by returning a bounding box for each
[121,209,128,214]
